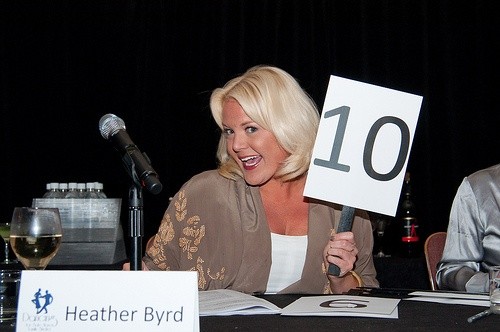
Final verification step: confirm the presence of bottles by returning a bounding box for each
[398,172,420,251]
[43,182,107,241]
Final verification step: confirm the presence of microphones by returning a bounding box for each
[99,113,162,195]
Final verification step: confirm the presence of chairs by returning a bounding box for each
[424,232,446,290]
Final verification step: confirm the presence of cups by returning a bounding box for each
[489,266,500,315]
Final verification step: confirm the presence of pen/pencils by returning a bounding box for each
[467,309,490,323]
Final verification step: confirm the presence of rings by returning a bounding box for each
[353,241,357,246]
[348,244,355,252]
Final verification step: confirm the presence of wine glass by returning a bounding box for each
[0,206,63,332]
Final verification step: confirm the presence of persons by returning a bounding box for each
[435,164,500,295]
[123,65,380,295]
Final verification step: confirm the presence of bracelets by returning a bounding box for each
[330,270,362,295]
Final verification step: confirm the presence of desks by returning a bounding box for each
[200,293,500,332]
